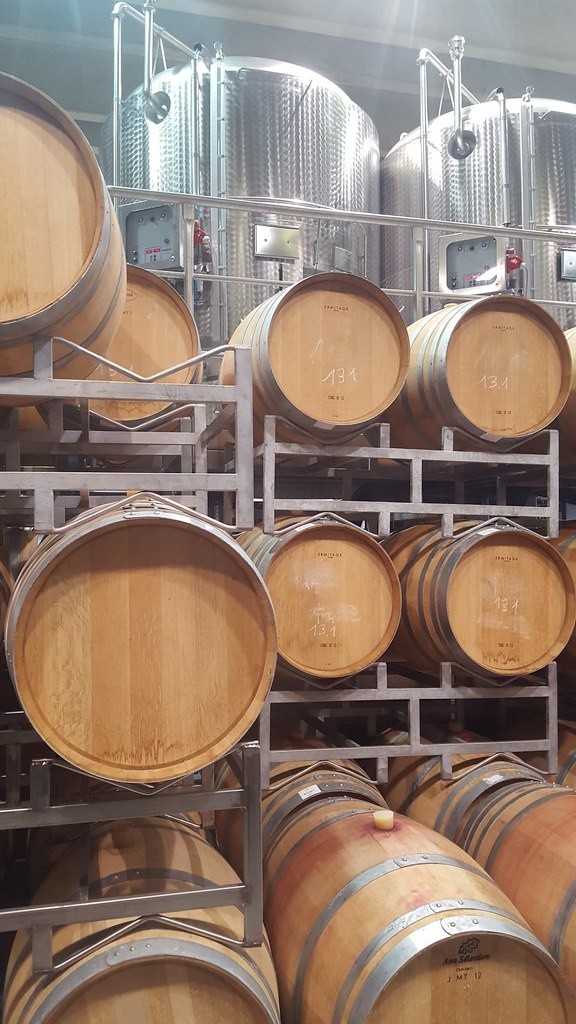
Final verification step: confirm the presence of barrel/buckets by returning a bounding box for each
[0,62,576,1024]
